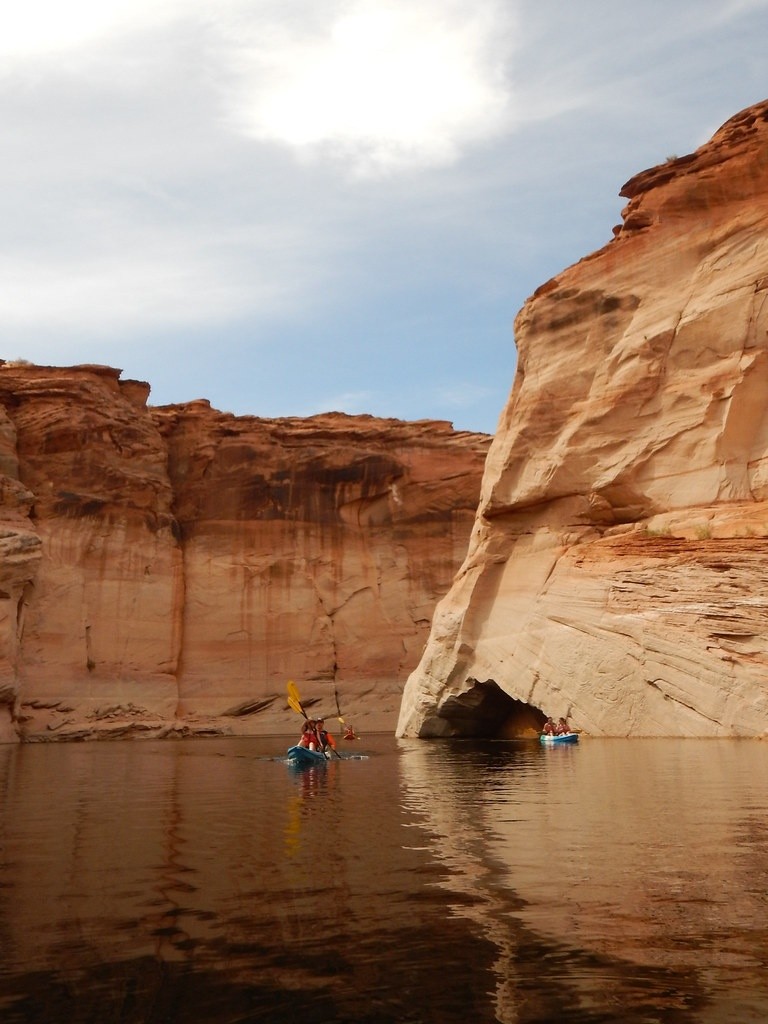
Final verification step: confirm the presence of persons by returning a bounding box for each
[297,716,336,759]
[557,717,570,736]
[344,725,356,736]
[542,717,558,736]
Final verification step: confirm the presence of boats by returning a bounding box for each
[288,745,331,762]
[540,733,579,741]
[344,734,355,740]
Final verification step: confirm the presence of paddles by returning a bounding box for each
[526,729,582,735]
[285,679,329,761]
[287,695,343,760]
[338,717,357,737]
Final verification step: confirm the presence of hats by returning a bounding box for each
[308,717,316,722]
[316,717,324,723]
